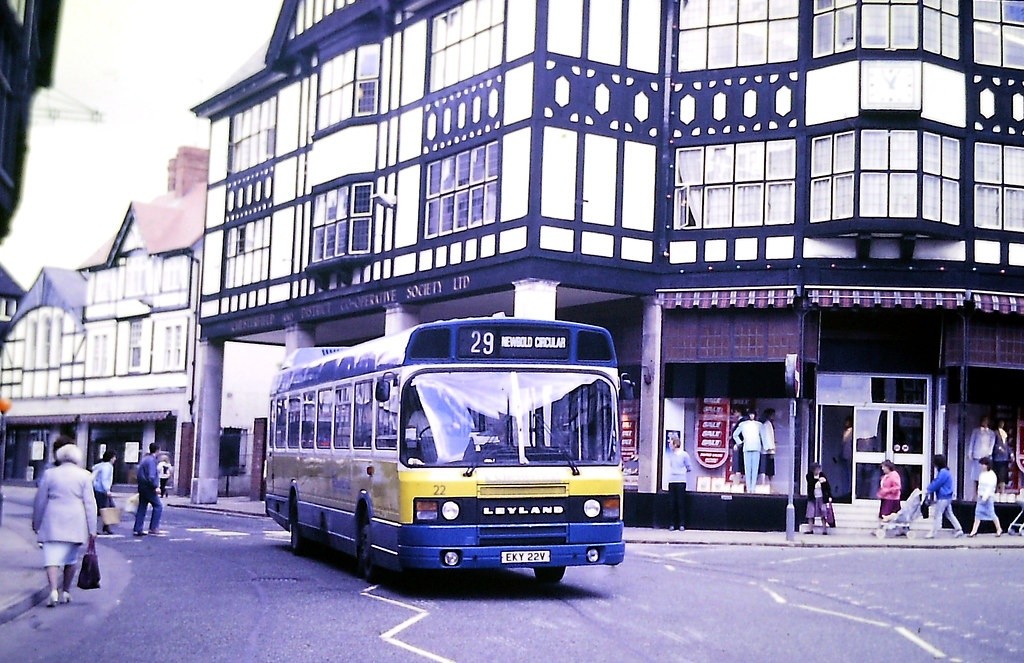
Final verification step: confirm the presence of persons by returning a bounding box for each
[872,459,905,537]
[92,450,117,534]
[967,457,1002,537]
[803,463,832,534]
[923,455,963,538]
[133,443,166,536]
[733,410,762,494]
[970,416,995,501]
[758,409,775,487]
[156,455,171,497]
[993,420,1015,487]
[410,402,433,437]
[36,436,75,486]
[667,437,692,531]
[733,406,746,477]
[32,444,97,607]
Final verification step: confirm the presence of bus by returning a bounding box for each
[263,314,637,586]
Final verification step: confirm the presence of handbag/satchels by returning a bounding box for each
[826,501,836,528]
[77,538,101,589]
[921,498,929,519]
[99,496,121,525]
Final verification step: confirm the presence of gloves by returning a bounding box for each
[819,477,826,483]
[829,497,832,502]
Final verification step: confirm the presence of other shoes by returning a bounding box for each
[133,531,147,536]
[804,530,813,534]
[38,540,43,549]
[924,533,935,539]
[954,530,963,538]
[148,530,166,537]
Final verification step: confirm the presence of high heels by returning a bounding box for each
[103,525,113,534]
[46,591,58,607]
[61,592,72,603]
[967,531,978,538]
[995,530,1003,537]
